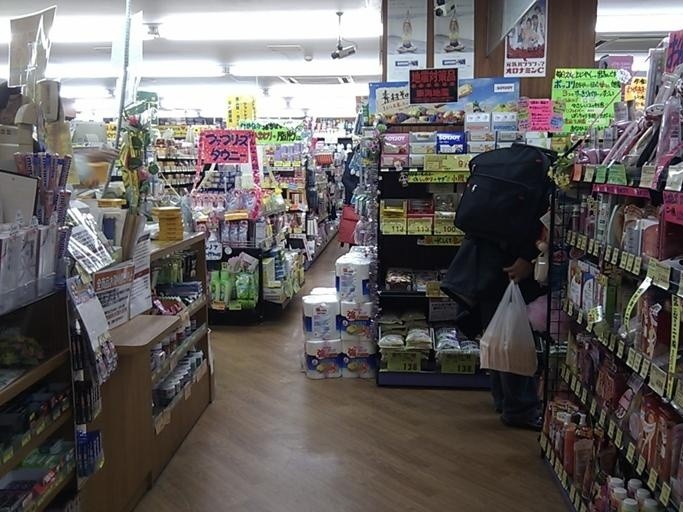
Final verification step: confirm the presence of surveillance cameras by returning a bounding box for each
[433,0,455,18]
[330,46,355,60]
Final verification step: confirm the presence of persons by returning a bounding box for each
[510,4,545,51]
[439,143,555,432]
[340,142,359,250]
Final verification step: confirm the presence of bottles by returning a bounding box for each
[607,478,659,512]
[402,19,413,47]
[448,16,458,45]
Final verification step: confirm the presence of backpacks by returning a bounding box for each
[453,145,551,252]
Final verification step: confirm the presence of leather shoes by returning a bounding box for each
[500,401,544,431]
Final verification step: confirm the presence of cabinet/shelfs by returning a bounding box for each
[540,137,683,512]
[374,122,550,391]
[0,170,76,511]
[69,232,215,512]
